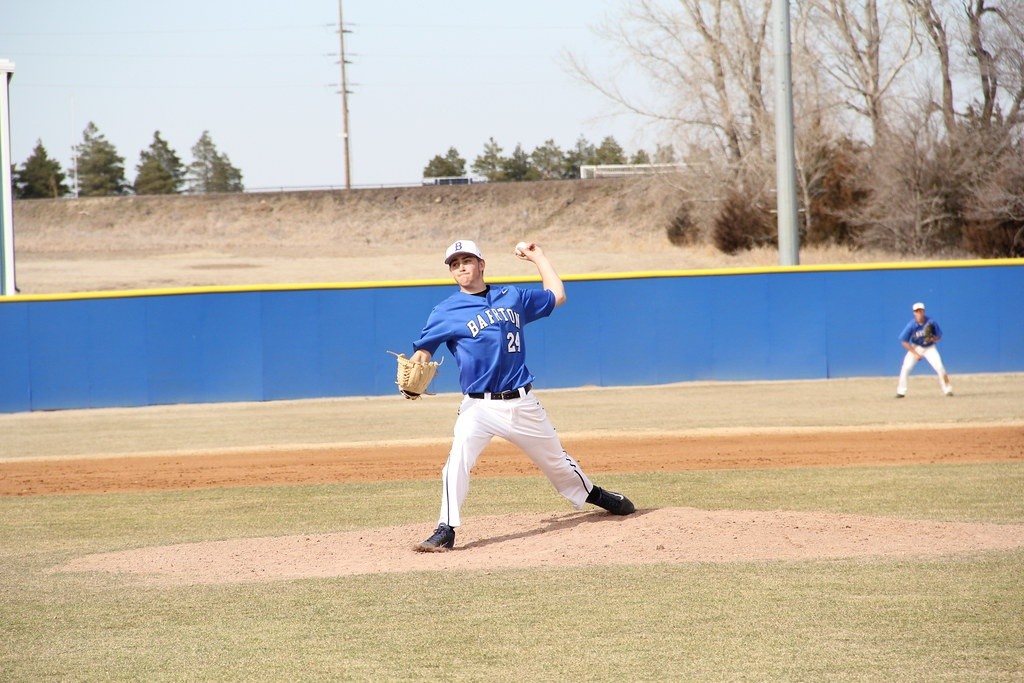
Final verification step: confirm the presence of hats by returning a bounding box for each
[444,240,482,266]
[913,303,924,311]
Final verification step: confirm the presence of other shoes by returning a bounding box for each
[947,392,953,396]
[898,394,904,398]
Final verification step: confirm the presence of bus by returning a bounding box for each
[421,175,473,186]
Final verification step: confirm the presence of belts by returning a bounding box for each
[469,383,532,399]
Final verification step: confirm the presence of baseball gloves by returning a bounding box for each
[396,354,438,400]
[923,323,933,343]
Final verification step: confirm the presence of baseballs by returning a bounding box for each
[516,242,527,255]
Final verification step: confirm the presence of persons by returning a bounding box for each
[396,241,635,554]
[896,302,954,397]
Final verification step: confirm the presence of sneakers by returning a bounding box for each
[420,523,456,549]
[587,486,634,515]
